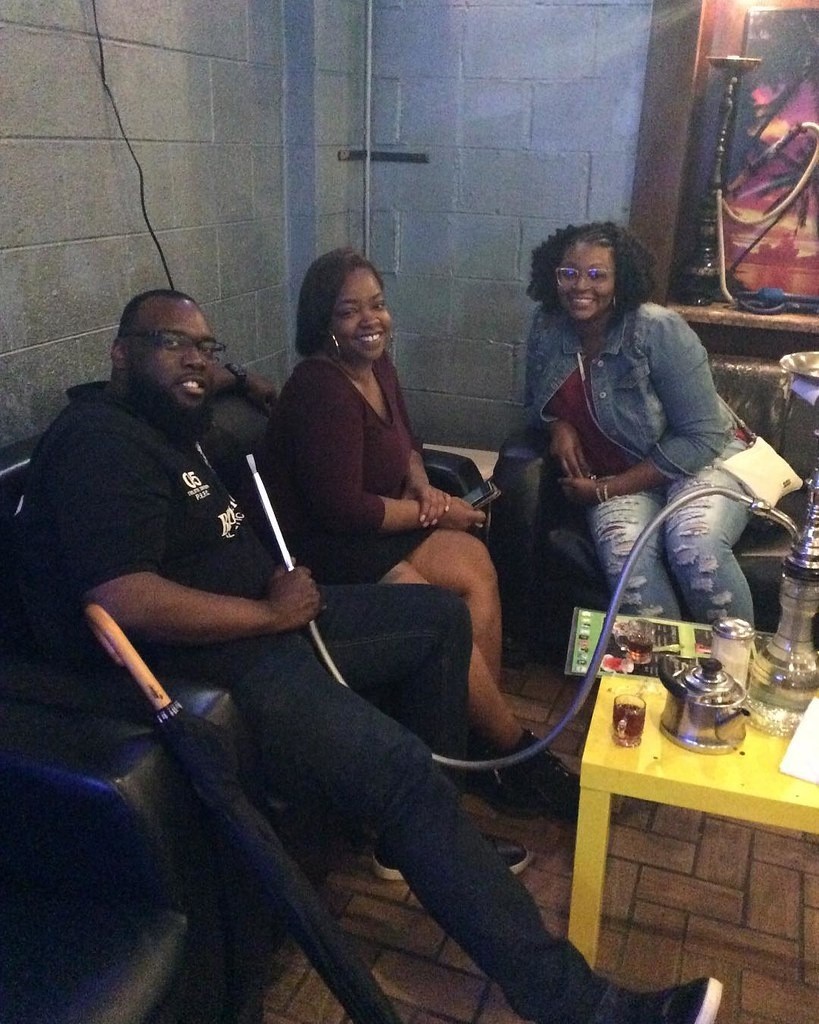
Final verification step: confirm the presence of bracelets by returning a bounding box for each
[595,479,608,503]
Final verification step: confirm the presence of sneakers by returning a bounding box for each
[371,826,531,883]
[587,977,731,1024]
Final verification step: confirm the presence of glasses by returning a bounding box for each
[116,330,228,364]
[554,266,618,290]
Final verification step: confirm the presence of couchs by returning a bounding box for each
[491,356,819,666]
[0,430,484,1023]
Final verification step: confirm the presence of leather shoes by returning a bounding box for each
[468,742,527,810]
[503,728,580,819]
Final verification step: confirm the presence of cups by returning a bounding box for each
[612,695,647,747]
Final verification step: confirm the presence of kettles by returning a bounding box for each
[659,653,751,754]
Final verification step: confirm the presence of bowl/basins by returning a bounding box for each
[780,351,819,382]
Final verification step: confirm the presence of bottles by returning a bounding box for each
[711,616,757,691]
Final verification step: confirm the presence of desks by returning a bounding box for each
[570,656,819,971]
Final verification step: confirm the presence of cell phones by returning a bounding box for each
[461,480,493,506]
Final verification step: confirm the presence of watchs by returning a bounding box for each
[224,362,249,396]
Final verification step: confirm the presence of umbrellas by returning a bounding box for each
[84,603,403,1024]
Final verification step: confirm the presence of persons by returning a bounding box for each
[11,288,725,1024]
[276,247,579,820]
[524,220,757,627]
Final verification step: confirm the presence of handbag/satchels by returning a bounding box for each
[707,432,804,509]
